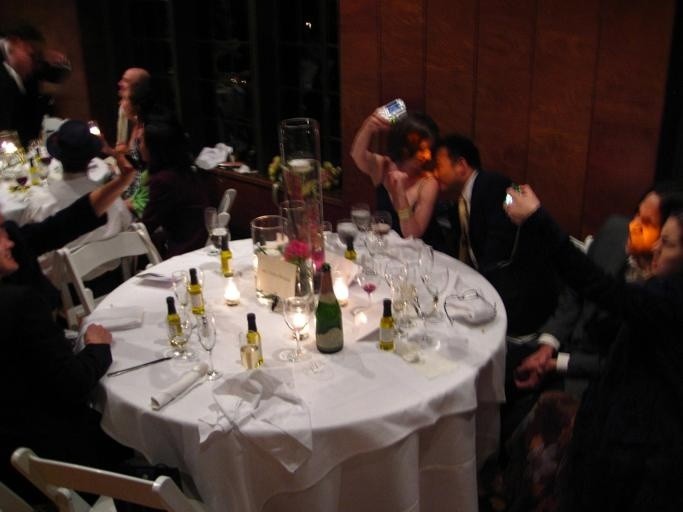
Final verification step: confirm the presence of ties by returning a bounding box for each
[458,196,479,267]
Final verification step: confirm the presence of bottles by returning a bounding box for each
[245,312,263,367]
[165,297,184,344]
[377,297,395,351]
[186,268,204,316]
[315,263,343,355]
[28,148,42,185]
[343,234,356,262]
[220,232,233,276]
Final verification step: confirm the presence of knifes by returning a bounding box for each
[106,356,177,378]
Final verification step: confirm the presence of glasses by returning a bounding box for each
[442,287,496,325]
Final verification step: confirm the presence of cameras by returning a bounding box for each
[379,98,408,120]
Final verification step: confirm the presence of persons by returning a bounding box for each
[432,132,557,333]
[0,122,152,512]
[501,186,683,427]
[3,36,216,259]
[348,98,441,239]
[492,180,682,512]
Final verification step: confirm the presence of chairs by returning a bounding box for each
[10,446,210,512]
[50,218,163,323]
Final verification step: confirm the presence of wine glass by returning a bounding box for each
[165,313,223,381]
[10,153,53,191]
[336,203,450,365]
[283,295,311,364]
[204,207,222,254]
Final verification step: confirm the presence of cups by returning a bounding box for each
[250,199,307,261]
[278,116,321,276]
[170,266,207,311]
[236,330,260,371]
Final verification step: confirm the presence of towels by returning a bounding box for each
[448,273,498,325]
[194,371,314,480]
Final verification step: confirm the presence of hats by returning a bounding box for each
[45,119,103,160]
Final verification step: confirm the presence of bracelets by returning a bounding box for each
[395,206,412,220]
